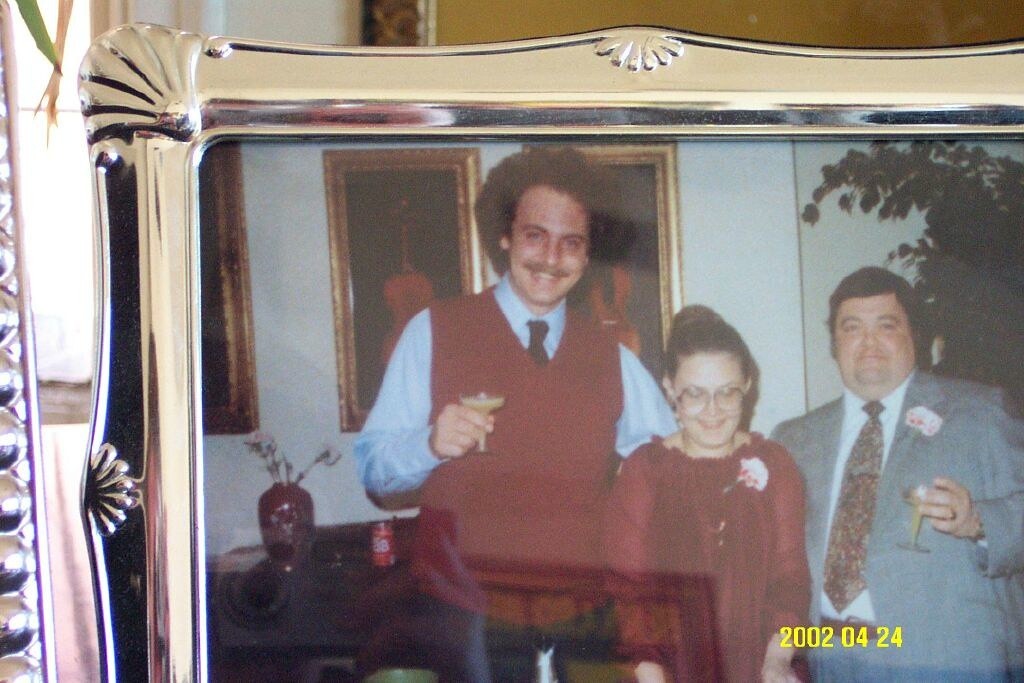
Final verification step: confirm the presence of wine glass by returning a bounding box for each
[896,460,949,555]
[458,374,509,459]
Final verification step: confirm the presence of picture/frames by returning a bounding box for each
[524,141,685,376]
[199,144,259,436]
[323,149,490,434]
[78,23,1024,683]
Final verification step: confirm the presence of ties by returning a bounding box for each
[824,400,886,615]
[526,318,549,365]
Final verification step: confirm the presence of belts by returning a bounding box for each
[820,616,879,641]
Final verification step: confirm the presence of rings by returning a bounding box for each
[949,506,956,520]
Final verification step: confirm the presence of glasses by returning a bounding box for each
[674,384,749,416]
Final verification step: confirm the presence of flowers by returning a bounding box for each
[905,405,942,443]
[721,457,770,494]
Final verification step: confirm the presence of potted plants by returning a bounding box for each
[244,439,342,576]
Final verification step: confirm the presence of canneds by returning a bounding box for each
[371,522,395,568]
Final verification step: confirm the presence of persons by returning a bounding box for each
[352,145,1023,683]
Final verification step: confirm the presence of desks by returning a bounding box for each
[208,517,414,683]
